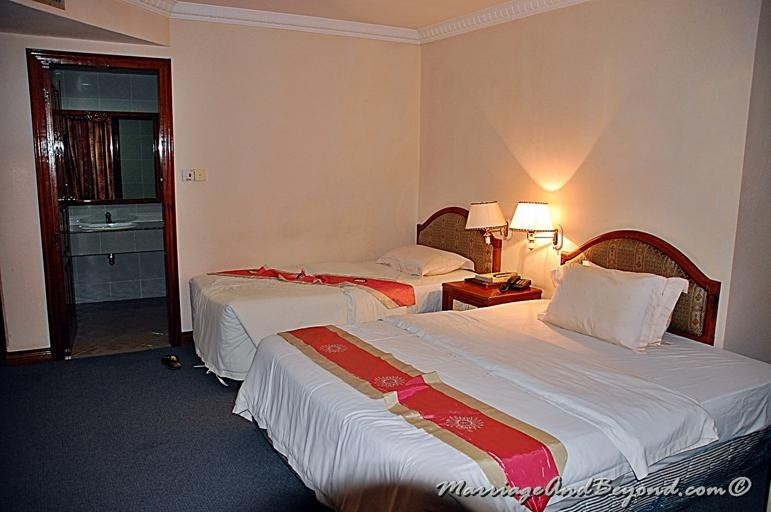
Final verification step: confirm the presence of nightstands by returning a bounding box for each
[439,278,533,312]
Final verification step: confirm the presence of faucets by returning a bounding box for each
[106,211,112,222]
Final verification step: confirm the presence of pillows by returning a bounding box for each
[582,258,688,350]
[374,243,468,278]
[541,260,649,354]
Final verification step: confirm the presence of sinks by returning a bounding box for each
[75,214,140,231]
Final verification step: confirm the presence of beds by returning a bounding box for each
[235,227,771,512]
[183,205,498,383]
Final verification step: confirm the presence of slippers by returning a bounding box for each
[160,353,182,369]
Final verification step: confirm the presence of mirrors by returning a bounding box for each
[59,109,158,205]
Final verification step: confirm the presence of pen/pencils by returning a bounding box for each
[493,273,512,278]
[353,280,367,282]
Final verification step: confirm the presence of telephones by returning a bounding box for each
[507,276,531,290]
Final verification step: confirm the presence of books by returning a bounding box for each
[465,271,517,287]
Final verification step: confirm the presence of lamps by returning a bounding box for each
[512,200,561,253]
[462,202,511,240]
